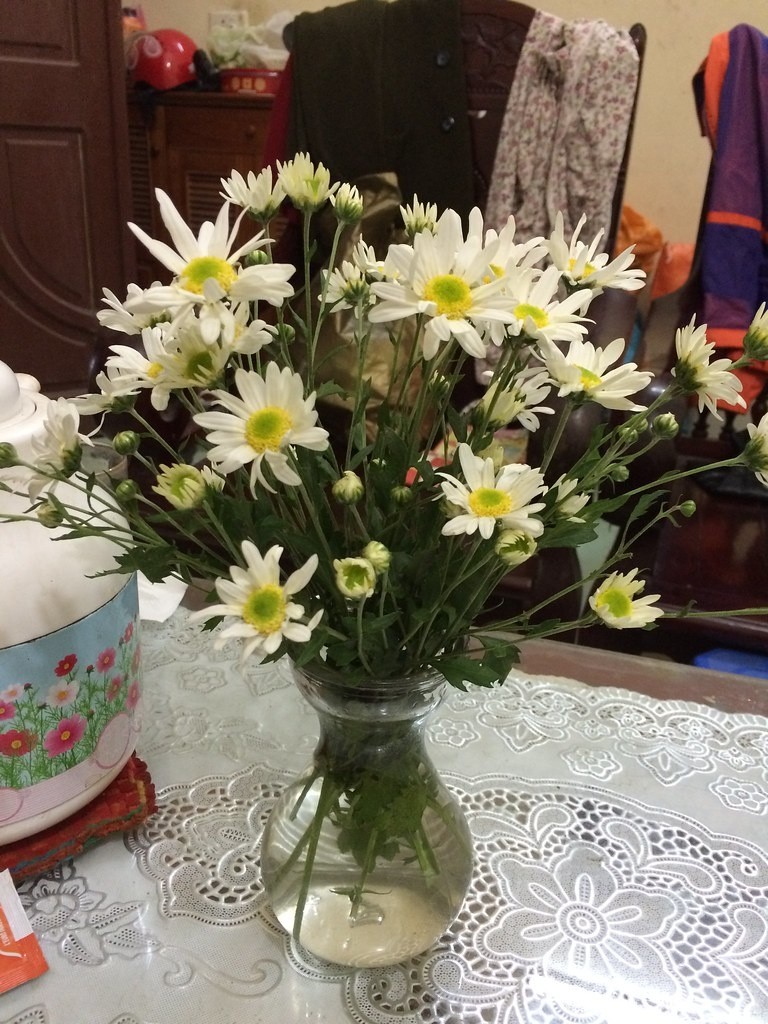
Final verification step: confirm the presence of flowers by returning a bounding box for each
[0,146,768,909]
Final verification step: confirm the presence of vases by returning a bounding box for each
[261,634,473,977]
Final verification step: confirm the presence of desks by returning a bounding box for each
[0,568,768,1024]
[125,88,292,318]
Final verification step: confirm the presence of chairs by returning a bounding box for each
[281,0,768,658]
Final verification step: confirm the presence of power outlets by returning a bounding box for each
[208,9,253,37]
